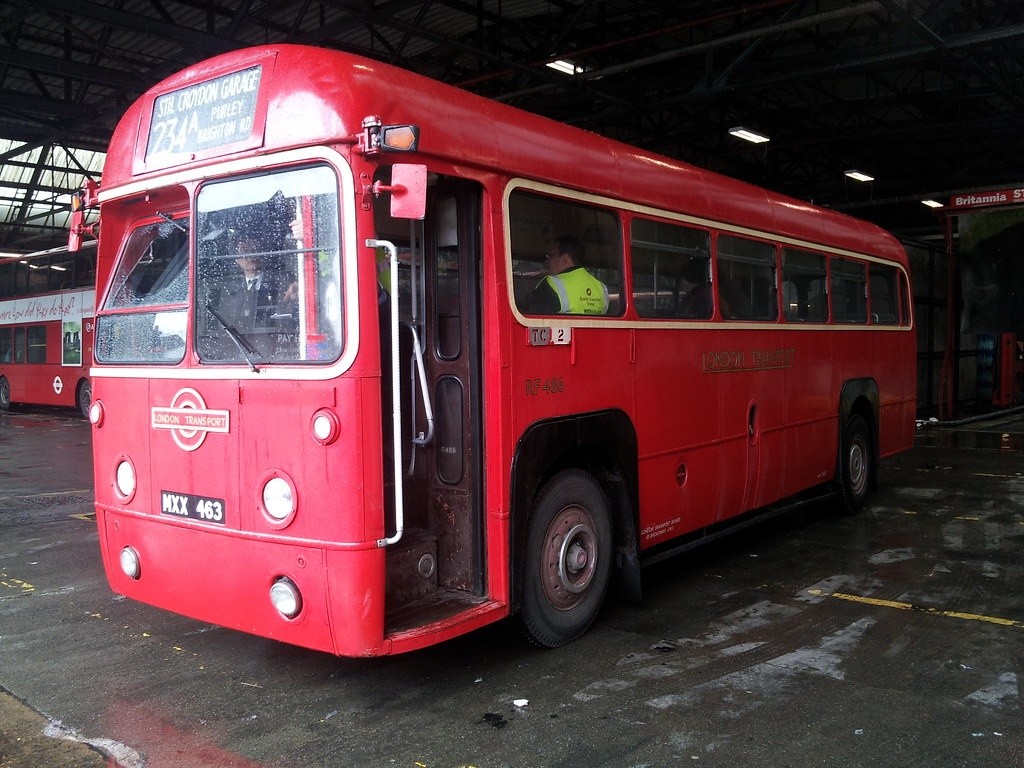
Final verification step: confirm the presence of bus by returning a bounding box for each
[68,43,921,659]
[0,238,98,420]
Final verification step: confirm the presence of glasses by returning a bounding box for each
[545,251,571,262]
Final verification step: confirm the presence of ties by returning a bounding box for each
[248,278,257,317]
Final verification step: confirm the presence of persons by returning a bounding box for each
[673,265,732,320]
[519,233,608,317]
[215,234,298,333]
